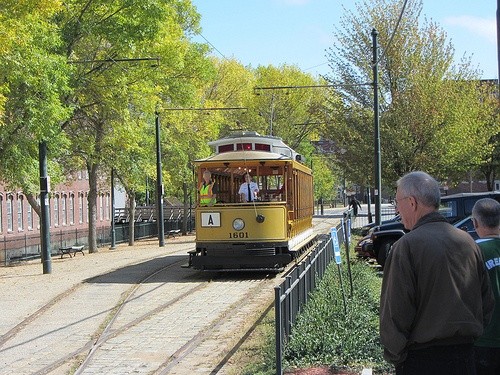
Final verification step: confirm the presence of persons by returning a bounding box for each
[472,198,500,375]
[238,173,259,202]
[197,171,217,206]
[349,197,362,216]
[379,172,495,375]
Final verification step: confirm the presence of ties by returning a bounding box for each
[247,184,251,201]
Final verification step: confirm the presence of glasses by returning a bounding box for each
[394,197,409,206]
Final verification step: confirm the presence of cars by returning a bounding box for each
[354,236,373,259]
[452,214,481,241]
[361,208,453,237]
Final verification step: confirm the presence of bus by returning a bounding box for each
[180,129,318,274]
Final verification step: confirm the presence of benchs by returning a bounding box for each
[167,229,181,239]
[58,245,85,259]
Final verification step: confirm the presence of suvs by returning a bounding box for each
[367,190,500,269]
